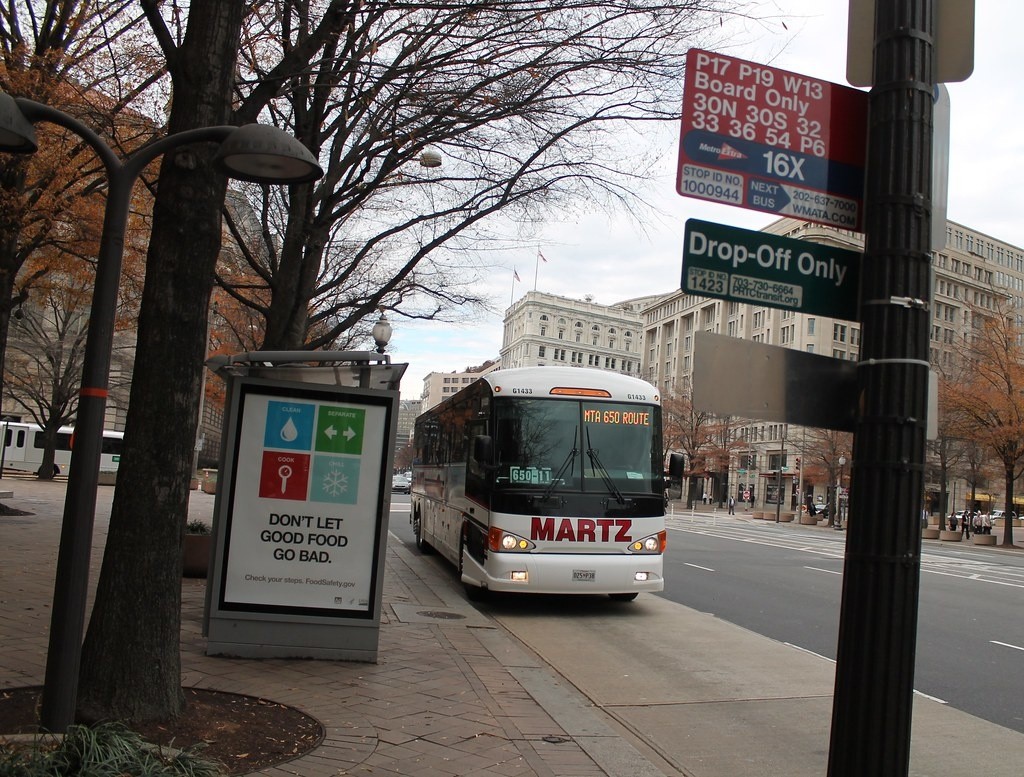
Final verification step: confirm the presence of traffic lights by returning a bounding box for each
[773,471,778,481]
[748,455,753,466]
[795,457,802,472]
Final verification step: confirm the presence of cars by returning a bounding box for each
[391,476,409,494]
[404,472,412,482]
[806,504,830,518]
[948,511,976,523]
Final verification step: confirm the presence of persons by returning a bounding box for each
[729,496,735,515]
[961,511,971,539]
[709,494,713,505]
[971,510,982,534]
[702,491,707,504]
[948,512,959,531]
[922,508,928,529]
[750,496,755,508]
[982,511,992,534]
[809,501,818,515]
[664,493,669,515]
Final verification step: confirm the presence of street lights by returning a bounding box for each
[0,417,13,480]
[190,266,232,490]
[834,455,845,531]
[243,325,259,354]
[20,93,328,734]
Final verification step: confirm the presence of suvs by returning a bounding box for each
[980,510,1017,521]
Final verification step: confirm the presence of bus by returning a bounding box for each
[410,365,686,603]
[0,421,125,479]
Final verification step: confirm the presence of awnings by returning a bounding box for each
[759,470,780,477]
[966,492,997,502]
[1012,496,1024,504]
